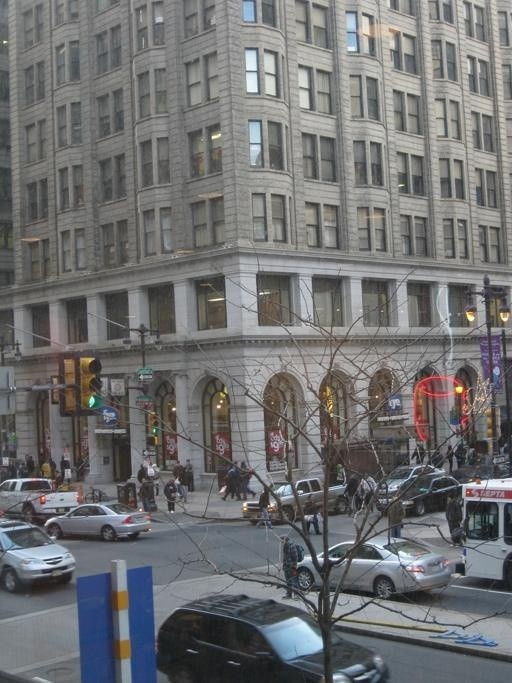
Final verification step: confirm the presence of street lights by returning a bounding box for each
[122,322,164,490]
[464,273,512,478]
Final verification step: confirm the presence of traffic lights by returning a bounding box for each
[57,355,104,419]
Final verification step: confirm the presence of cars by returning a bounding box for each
[0,519,77,594]
[240,461,512,526]
[43,500,153,542]
[290,536,453,600]
[155,593,390,683]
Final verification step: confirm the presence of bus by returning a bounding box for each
[461,478,512,591]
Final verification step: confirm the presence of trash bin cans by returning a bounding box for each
[117,483,137,509]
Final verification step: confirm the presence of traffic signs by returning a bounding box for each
[135,395,153,407]
[135,368,153,384]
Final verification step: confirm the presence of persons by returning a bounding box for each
[255,484,274,528]
[218,460,257,500]
[382,496,403,539]
[454,442,466,466]
[279,535,304,599]
[301,493,322,534]
[431,450,444,468]
[447,445,454,473]
[445,494,462,548]
[411,443,425,463]
[5,454,70,483]
[335,461,378,518]
[138,458,193,512]
[466,443,475,464]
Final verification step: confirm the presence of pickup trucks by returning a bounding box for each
[0,477,80,525]
